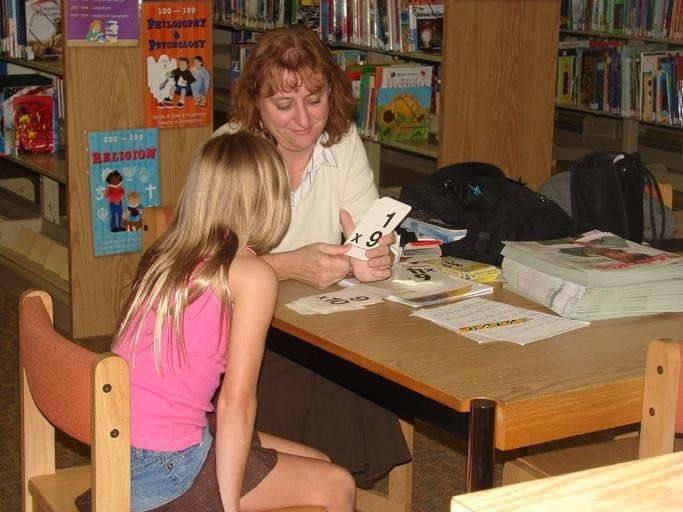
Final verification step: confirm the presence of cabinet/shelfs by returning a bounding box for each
[0,0,214,340]
[211,0,563,193]
[552,0,682,212]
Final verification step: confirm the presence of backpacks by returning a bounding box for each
[395,160,568,269]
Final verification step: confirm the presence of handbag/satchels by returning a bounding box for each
[570,150,666,248]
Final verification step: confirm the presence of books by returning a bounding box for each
[559,0,683,40]
[228,30,269,104]
[0,0,63,63]
[0,70,63,157]
[327,47,441,144]
[337,260,494,310]
[211,1,321,40]
[555,31,682,130]
[499,227,683,322]
[0,159,68,284]
[320,0,443,54]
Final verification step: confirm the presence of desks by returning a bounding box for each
[450,450,683,512]
[262,250,683,491]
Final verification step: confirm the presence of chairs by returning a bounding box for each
[18,287,328,512]
[645,183,673,247]
[141,206,415,512]
[502,337,683,487]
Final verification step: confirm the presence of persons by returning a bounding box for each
[209,29,412,490]
[73,117,355,510]
